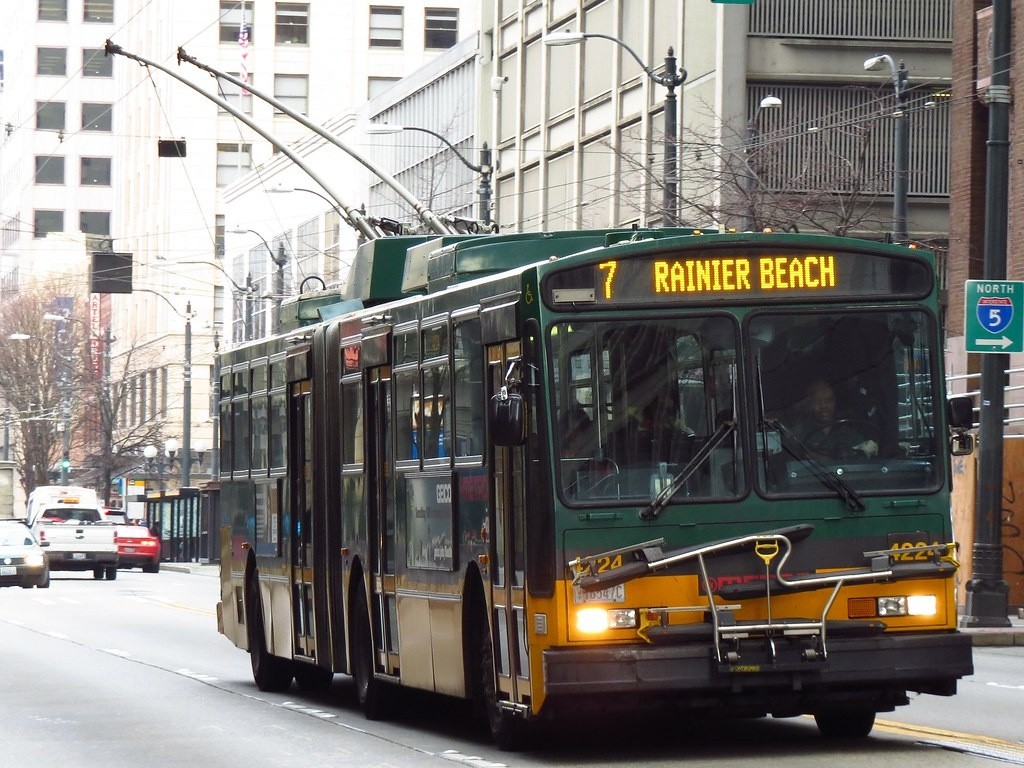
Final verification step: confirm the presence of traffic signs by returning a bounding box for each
[963,278,1024,354]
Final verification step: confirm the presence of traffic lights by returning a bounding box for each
[62,450,70,471]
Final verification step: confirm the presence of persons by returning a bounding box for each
[782,379,883,464]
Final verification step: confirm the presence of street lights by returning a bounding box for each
[361,120,495,234]
[544,30,689,227]
[166,438,208,488]
[864,54,910,246]
[164,259,260,341]
[43,313,118,508]
[144,446,179,490]
[272,184,368,249]
[743,93,781,233]
[230,225,289,335]
[132,289,194,488]
[9,332,72,485]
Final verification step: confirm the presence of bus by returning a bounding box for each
[101,34,974,751]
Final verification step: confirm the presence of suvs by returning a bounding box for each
[102,509,133,525]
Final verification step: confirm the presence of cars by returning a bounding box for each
[0,518,51,588]
[115,523,161,573]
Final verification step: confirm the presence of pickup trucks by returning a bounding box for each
[28,503,119,581]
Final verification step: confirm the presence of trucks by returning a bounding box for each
[24,485,99,527]
